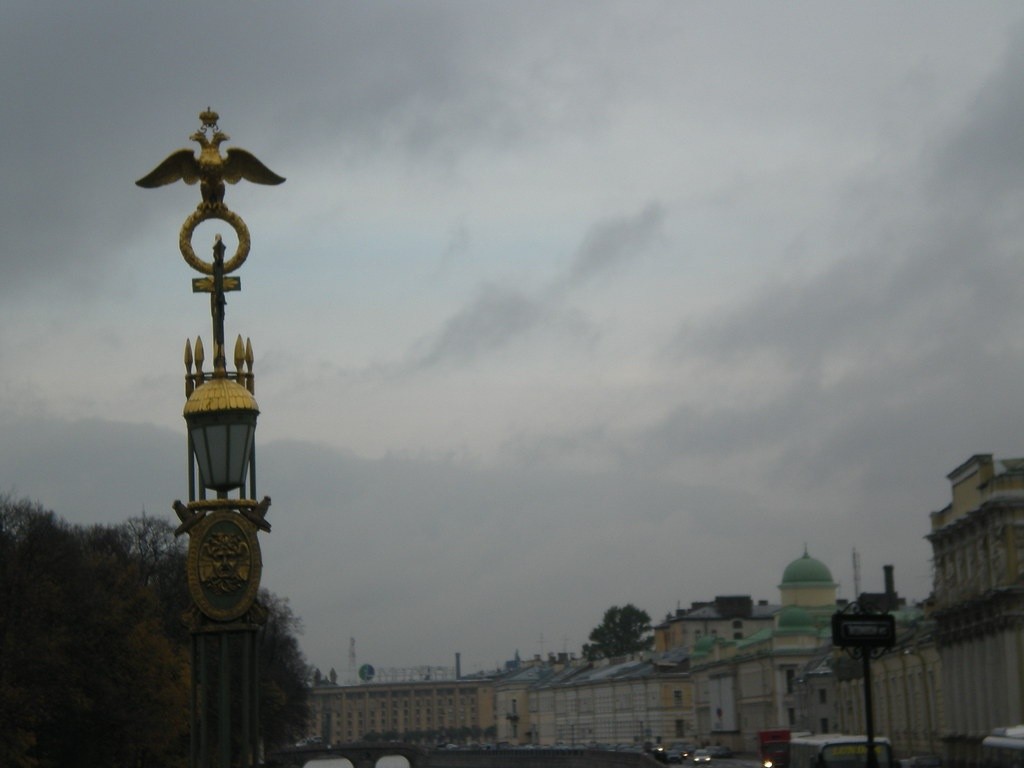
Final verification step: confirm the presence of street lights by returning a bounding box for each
[135,105,285,768]
[906,650,936,753]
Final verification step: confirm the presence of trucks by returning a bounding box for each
[757,728,791,768]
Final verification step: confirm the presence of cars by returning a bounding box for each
[295,735,326,750]
[434,742,732,766]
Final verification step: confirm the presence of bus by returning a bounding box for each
[790,735,893,768]
[982,725,1024,768]
